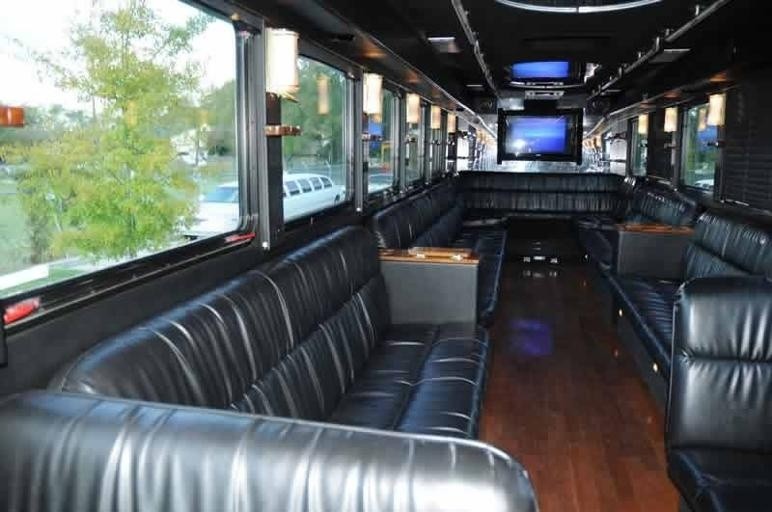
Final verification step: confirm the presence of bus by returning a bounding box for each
[380,139,419,173]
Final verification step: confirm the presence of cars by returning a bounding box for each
[342,172,393,200]
[170,169,347,244]
[691,178,714,197]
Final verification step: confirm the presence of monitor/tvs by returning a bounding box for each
[498,108,583,165]
[504,58,586,88]
